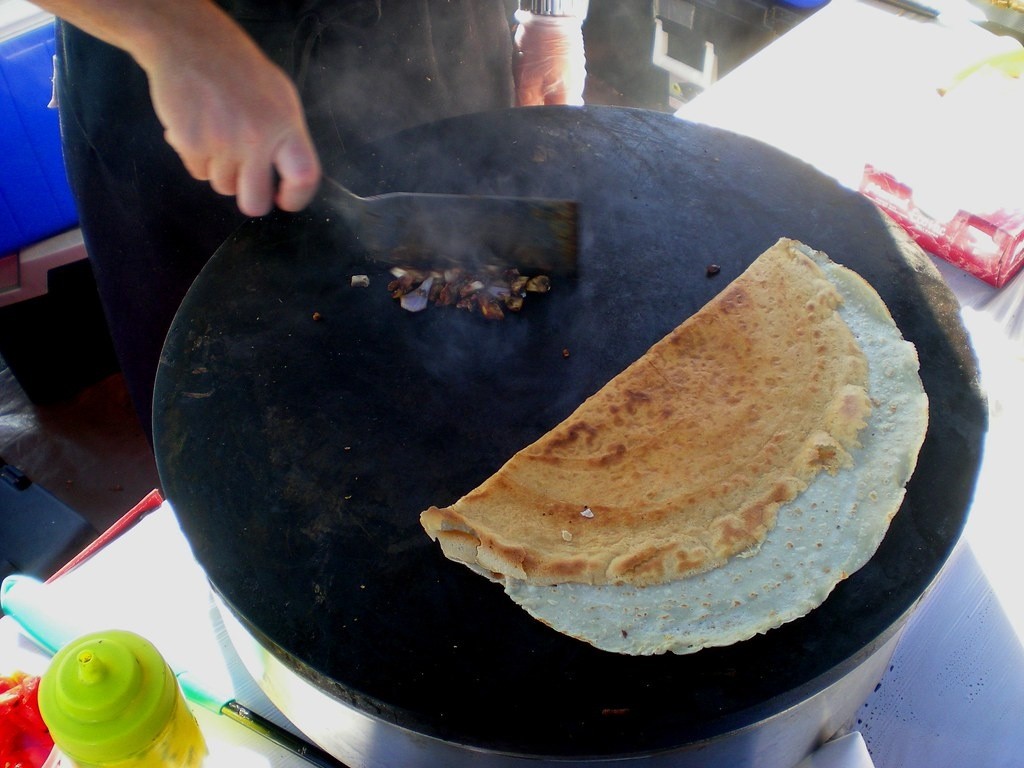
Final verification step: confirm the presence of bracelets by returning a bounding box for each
[519,0,589,17]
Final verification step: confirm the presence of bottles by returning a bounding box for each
[36,629,207,768]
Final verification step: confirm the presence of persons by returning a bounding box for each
[27,0,592,462]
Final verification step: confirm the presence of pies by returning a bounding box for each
[420,236,930,656]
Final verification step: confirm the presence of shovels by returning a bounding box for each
[881,0,1024,49]
[269,166,582,296]
